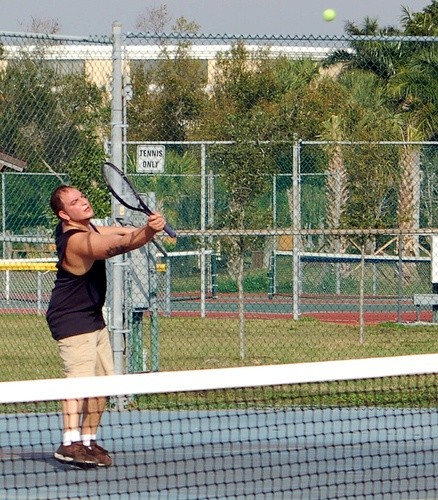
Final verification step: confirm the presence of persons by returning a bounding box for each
[46,185,166,468]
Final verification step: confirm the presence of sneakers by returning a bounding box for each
[54,441,98,467]
[82,440,112,467]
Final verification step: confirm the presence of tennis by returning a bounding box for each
[323,9,335,22]
